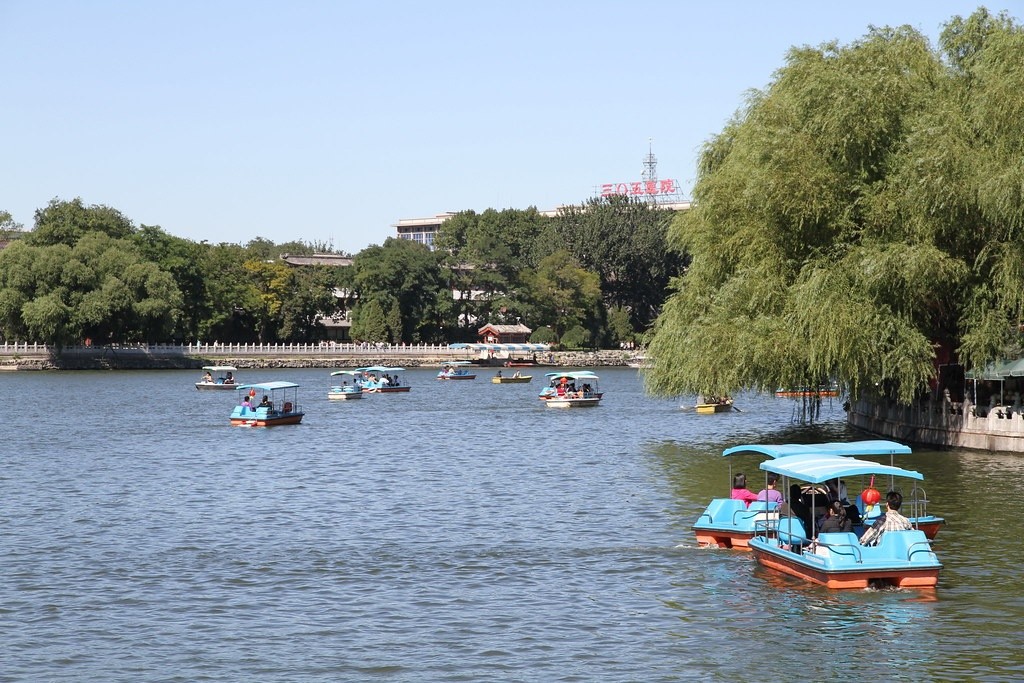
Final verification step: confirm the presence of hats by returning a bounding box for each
[829,500,843,514]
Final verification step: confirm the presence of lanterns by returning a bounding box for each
[862,489,879,511]
[560,377,567,383]
[250,391,255,397]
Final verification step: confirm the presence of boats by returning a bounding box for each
[327,370,364,399]
[691,439,946,552]
[437,361,477,380]
[539,370,604,399]
[355,367,411,393]
[747,454,946,594]
[229,381,306,427]
[504,354,540,368]
[492,375,534,384]
[195,365,247,390]
[546,375,599,408]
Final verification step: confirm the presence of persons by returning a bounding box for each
[756,470,782,515]
[357,368,401,387]
[513,370,521,378]
[441,366,448,374]
[202,371,212,383]
[860,490,913,549]
[810,502,851,552]
[352,379,359,392]
[730,475,758,510]
[257,396,272,411]
[343,380,347,386]
[496,370,501,378]
[447,367,456,375]
[240,396,251,412]
[847,504,864,538]
[826,476,850,509]
[781,485,812,542]
[223,372,233,385]
[546,378,597,401]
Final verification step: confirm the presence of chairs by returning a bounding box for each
[281,403,293,414]
[776,516,809,553]
